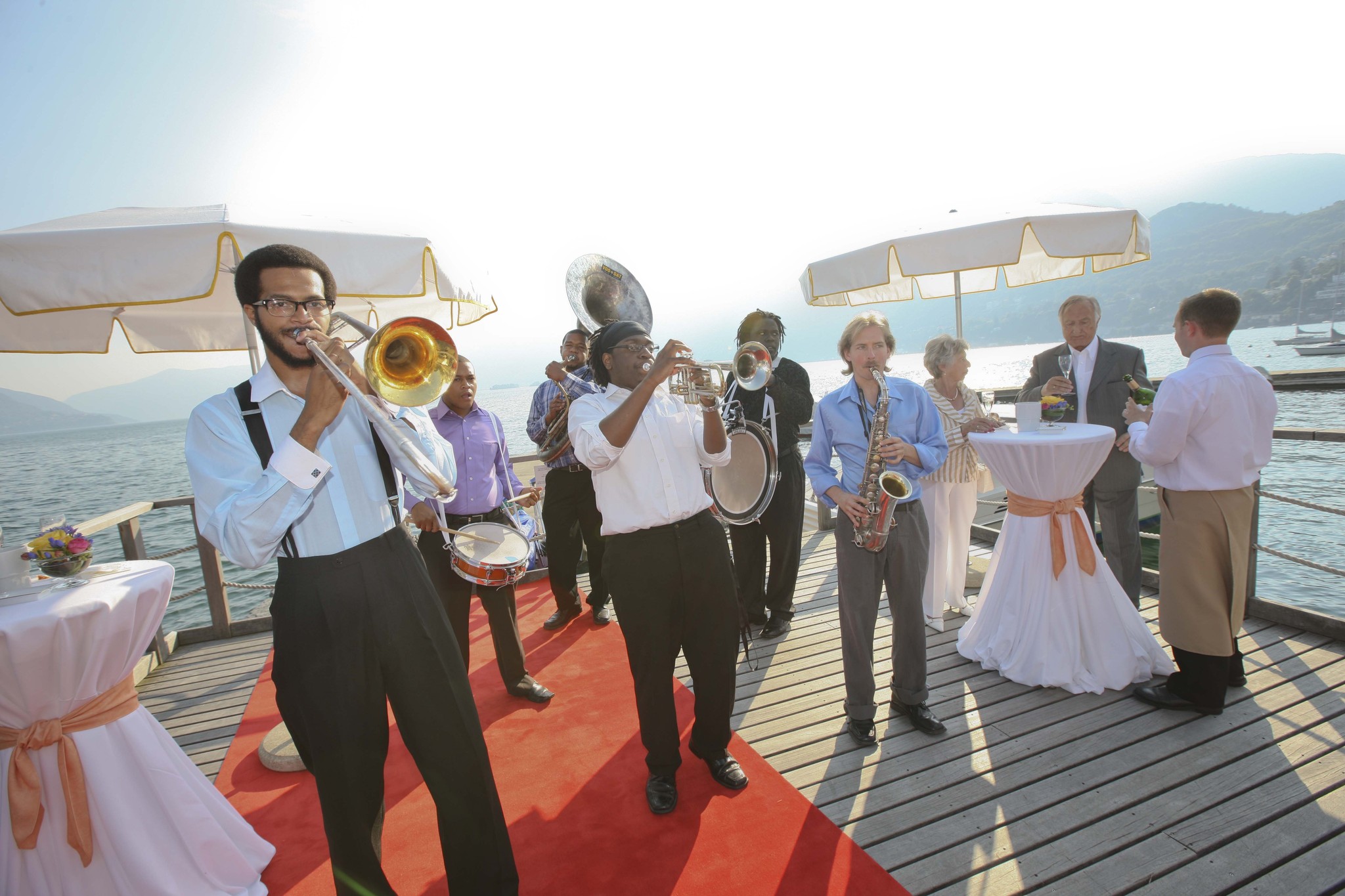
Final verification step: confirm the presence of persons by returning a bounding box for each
[567,319,758,814]
[720,308,814,639]
[185,244,519,896]
[526,329,612,630]
[1121,288,1279,715]
[1014,295,1155,611]
[917,334,1006,632]
[401,354,555,703]
[803,309,949,745]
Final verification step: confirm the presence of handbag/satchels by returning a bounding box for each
[977,462,994,493]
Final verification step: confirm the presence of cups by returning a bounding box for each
[0,545,33,592]
[39,514,67,531]
[0,525,2,550]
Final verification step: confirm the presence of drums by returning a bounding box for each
[700,419,779,527]
[450,522,531,586]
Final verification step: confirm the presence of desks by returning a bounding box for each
[956,422,1180,695]
[0,559,276,896]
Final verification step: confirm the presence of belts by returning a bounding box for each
[452,508,500,525]
[551,463,587,472]
[893,500,917,511]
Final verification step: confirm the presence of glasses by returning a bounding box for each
[606,343,659,352]
[253,299,335,317]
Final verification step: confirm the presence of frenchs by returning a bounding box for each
[536,253,653,463]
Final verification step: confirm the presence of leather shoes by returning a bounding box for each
[747,612,767,624]
[890,698,947,734]
[960,604,974,617]
[543,605,583,630]
[847,717,879,745]
[1133,685,1223,715]
[924,614,944,633]
[590,604,612,624]
[705,752,749,790]
[1226,675,1247,687]
[525,680,555,703]
[645,770,678,814]
[759,616,790,638]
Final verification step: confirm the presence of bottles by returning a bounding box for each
[1122,374,1158,410]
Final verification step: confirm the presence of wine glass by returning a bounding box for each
[1058,354,1076,396]
[981,392,996,433]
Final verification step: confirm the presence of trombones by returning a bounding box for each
[292,310,458,498]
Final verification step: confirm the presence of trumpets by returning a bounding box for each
[642,340,773,405]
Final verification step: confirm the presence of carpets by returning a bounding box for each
[214,576,914,896]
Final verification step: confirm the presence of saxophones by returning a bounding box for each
[849,366,913,553]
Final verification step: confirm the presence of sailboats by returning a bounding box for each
[1272,276,1330,346]
[1293,247,1345,355]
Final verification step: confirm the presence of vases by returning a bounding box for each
[33,546,90,591]
[1035,408,1066,430]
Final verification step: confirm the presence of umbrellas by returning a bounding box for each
[0,205,502,382]
[799,208,1150,333]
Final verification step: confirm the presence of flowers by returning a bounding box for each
[1038,396,1075,420]
[20,524,94,577]
